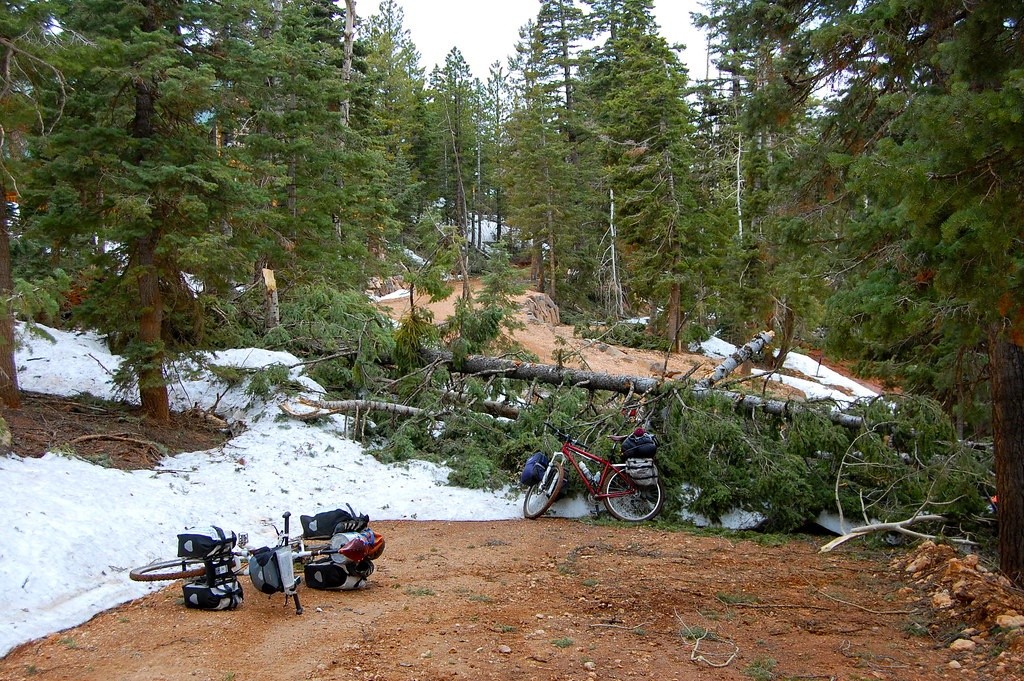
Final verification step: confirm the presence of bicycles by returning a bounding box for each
[129,509,385,616]
[523,420,665,522]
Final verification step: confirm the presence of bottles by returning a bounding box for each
[578,461,593,480]
[590,470,601,488]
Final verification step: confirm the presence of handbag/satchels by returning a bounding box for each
[176,524,237,557]
[299,503,384,590]
[181,578,243,610]
[624,427,660,488]
[546,467,570,503]
[522,452,549,485]
